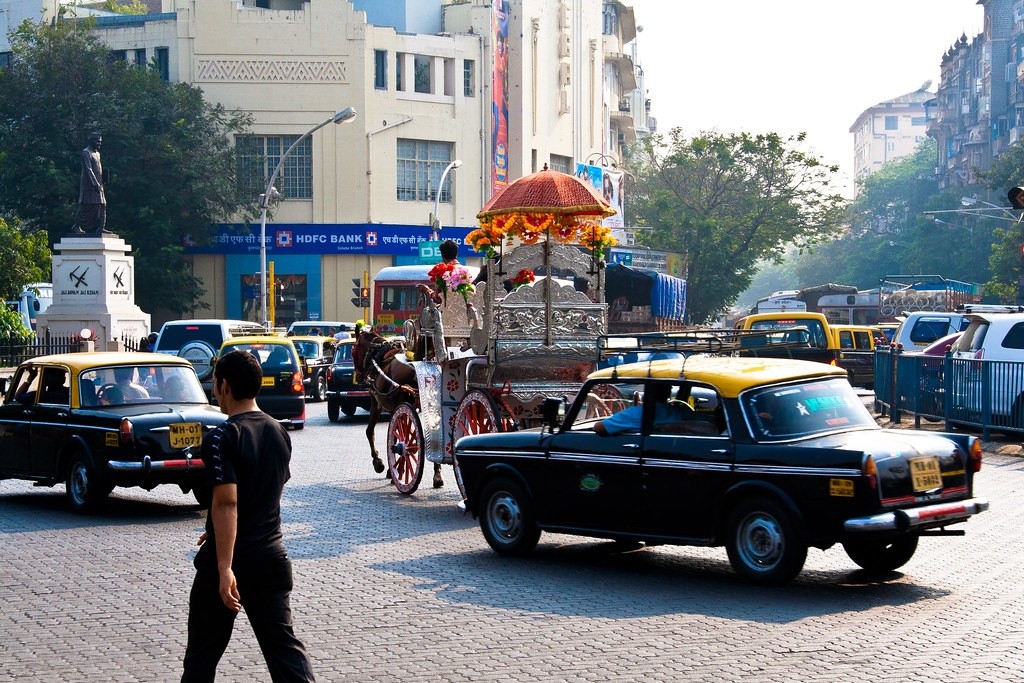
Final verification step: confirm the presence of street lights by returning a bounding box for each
[430,159,465,241]
[259,106,358,325]
[890,241,922,274]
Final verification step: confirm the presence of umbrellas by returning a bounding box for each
[477,161,618,346]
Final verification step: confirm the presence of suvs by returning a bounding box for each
[154,318,272,393]
[888,303,1024,444]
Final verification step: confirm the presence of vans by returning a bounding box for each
[732,312,839,369]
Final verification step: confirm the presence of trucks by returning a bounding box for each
[605,261,687,333]
[757,273,973,326]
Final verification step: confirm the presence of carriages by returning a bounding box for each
[349,163,618,508]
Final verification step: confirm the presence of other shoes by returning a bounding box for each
[405,351,416,361]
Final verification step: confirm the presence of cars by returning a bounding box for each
[451,356,989,596]
[210,335,306,432]
[0,350,228,519]
[790,322,901,391]
[283,320,376,423]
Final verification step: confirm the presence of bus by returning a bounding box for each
[372,265,481,338]
[3,282,54,345]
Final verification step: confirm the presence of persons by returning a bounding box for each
[105,367,150,401]
[610,295,626,320]
[604,173,623,214]
[405,239,472,362]
[274,274,306,326]
[16,361,69,404]
[137,332,158,352]
[288,325,351,343]
[71,132,112,235]
[495,31,505,77]
[594,383,695,433]
[179,351,317,683]
[579,165,591,186]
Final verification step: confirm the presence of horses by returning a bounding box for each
[352,323,444,489]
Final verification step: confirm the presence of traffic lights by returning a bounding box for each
[351,278,361,308]
[360,287,370,307]
[1007,186,1024,209]
